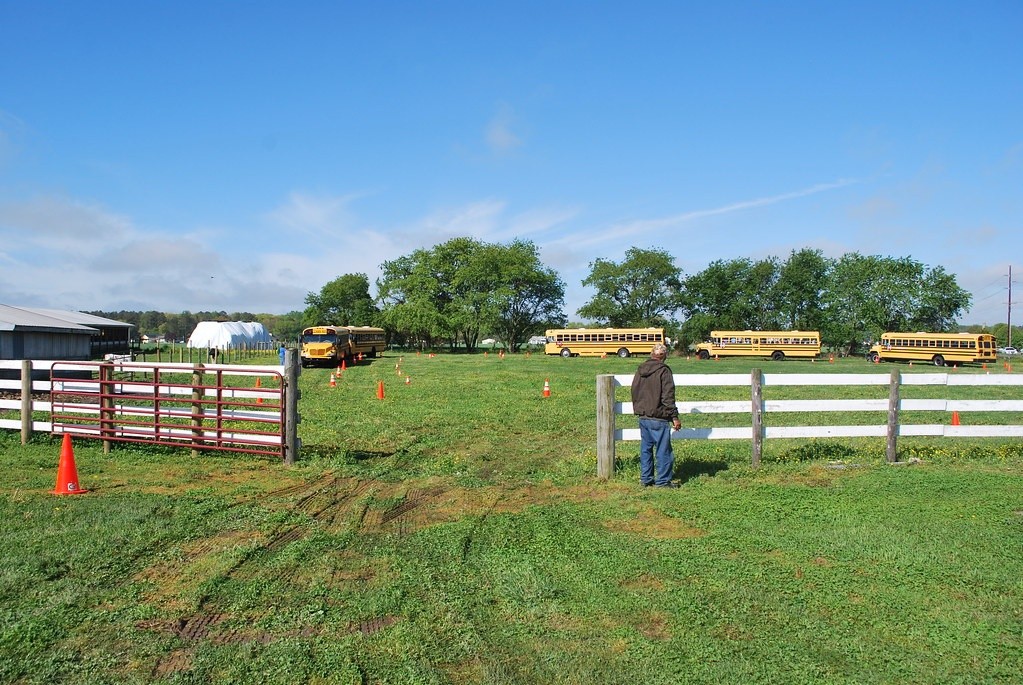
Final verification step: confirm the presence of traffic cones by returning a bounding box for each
[874,356,880,365]
[395,350,436,385]
[951,411,959,426]
[484,349,530,359]
[715,354,719,360]
[908,361,913,368]
[829,354,834,363]
[379,352,383,358]
[1003,361,1007,368]
[377,381,384,399]
[685,355,690,361]
[341,360,346,371]
[601,353,606,358]
[352,357,357,364]
[46,433,90,495]
[255,398,264,405]
[336,366,342,377]
[273,376,277,381]
[358,353,363,362]
[1007,365,1011,372]
[982,363,987,370]
[542,378,551,397]
[951,364,957,370]
[254,377,260,387]
[330,372,336,386]
[811,358,815,363]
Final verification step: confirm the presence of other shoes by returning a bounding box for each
[641,483,649,487]
[661,482,681,489]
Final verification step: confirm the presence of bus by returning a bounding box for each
[299,325,386,368]
[543,327,666,358]
[866,331,994,367]
[694,328,822,361]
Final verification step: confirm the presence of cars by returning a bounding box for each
[1002,347,1018,355]
[995,345,1003,353]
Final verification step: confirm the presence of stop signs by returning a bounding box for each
[557,342,562,347]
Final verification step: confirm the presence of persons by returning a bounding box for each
[630,344,683,488]
[278,343,287,365]
[723,339,816,343]
